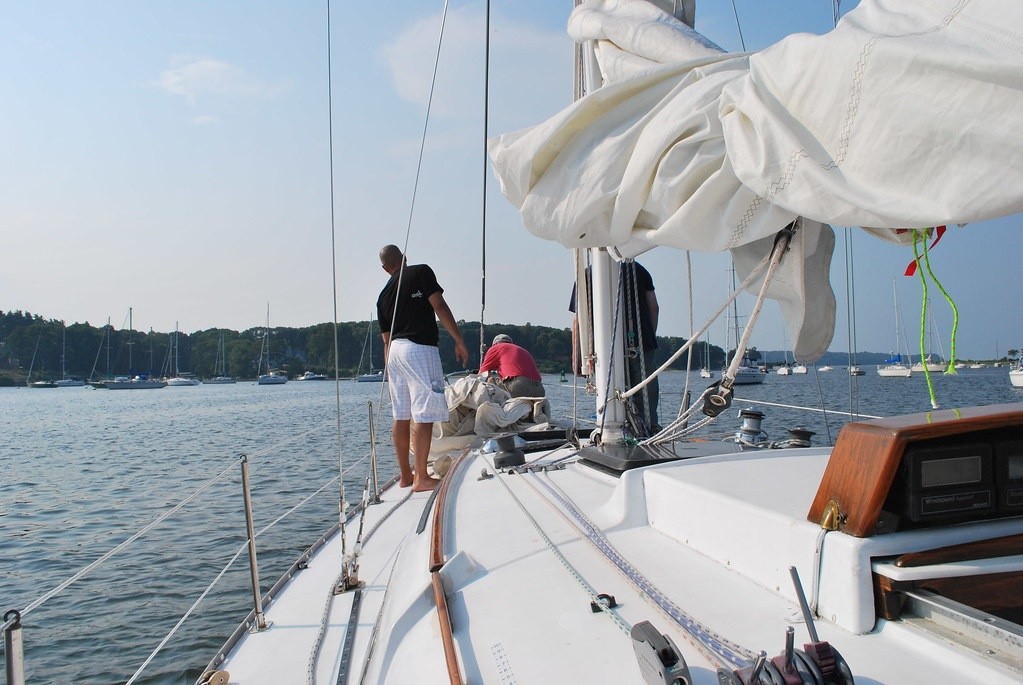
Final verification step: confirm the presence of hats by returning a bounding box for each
[493,334,513,345]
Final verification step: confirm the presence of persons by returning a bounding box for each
[377,245,468,492]
[478,335,545,398]
[569,261,663,437]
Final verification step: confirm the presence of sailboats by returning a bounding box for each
[18,301,387,390]
[699,255,1023,389]
